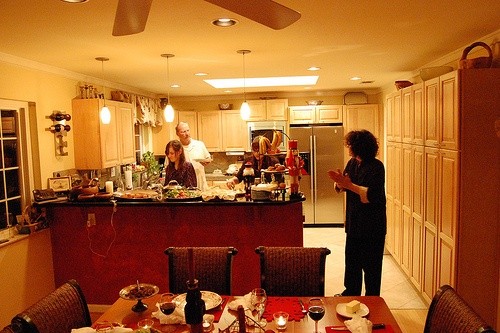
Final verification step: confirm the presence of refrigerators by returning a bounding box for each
[287,125,345,225]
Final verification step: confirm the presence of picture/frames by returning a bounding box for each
[48,176,72,192]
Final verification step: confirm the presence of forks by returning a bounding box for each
[299,300,307,313]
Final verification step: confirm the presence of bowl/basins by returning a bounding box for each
[218,103,234,110]
[76,184,112,201]
[306,101,323,105]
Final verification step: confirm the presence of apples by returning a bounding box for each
[279,183,286,188]
[268,163,285,171]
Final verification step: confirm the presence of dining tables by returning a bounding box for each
[93,291,403,333]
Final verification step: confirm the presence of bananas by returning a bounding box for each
[259,136,271,155]
[271,131,282,148]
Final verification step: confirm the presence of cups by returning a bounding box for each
[95,312,288,333]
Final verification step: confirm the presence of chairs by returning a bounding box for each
[164,246,238,295]
[424,284,496,333]
[256,247,331,297]
[0,279,92,333]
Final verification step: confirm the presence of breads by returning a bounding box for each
[120,192,150,198]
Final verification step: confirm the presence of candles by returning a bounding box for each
[125,171,132,189]
[105,180,113,194]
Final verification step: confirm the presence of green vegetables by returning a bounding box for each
[166,190,200,198]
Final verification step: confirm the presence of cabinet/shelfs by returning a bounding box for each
[71,98,135,170]
[151,98,381,155]
[382,67,500,328]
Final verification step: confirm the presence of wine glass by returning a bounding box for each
[251,288,267,327]
[308,298,326,333]
[160,293,177,333]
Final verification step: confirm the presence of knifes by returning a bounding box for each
[330,323,385,330]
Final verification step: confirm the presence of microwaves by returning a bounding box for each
[248,122,287,151]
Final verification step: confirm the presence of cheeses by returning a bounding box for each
[345,300,360,314]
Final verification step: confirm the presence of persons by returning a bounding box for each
[327,129,387,297]
[226,135,283,194]
[163,140,197,188]
[163,122,213,190]
[52,181,63,189]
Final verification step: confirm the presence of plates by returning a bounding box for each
[337,303,370,318]
[113,188,246,201]
[171,291,223,314]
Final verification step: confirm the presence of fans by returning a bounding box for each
[112,0,301,37]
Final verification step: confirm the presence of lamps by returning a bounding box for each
[95,57,110,123]
[161,54,174,123]
[237,50,251,119]
